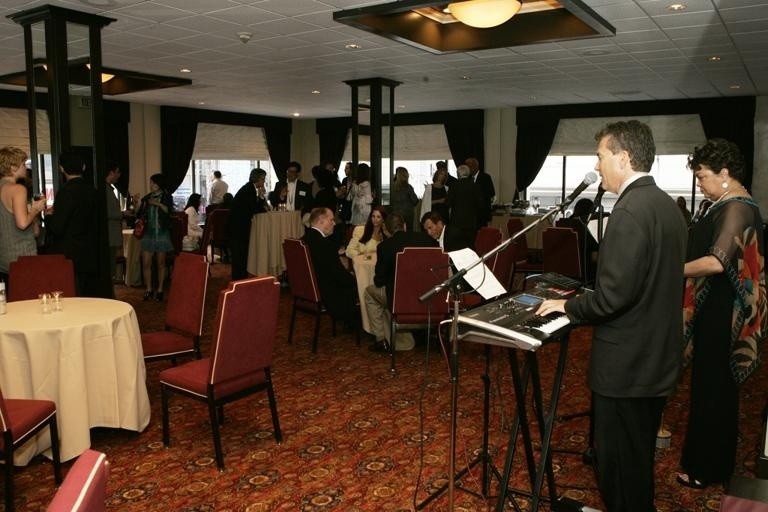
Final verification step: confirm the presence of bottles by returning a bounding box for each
[130,196,135,213]
[0,277,7,314]
[51,292,63,311]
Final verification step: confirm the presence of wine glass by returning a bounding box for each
[534,199,540,215]
[555,196,561,209]
[34,193,40,201]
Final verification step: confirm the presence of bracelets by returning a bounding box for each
[31,205,41,212]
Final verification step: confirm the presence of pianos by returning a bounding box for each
[450,271,594,352]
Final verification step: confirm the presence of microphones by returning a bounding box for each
[559,172,599,214]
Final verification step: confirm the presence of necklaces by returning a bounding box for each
[703,184,747,215]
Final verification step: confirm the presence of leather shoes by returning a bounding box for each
[368,339,389,352]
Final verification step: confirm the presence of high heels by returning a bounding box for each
[155,292,163,301]
[144,290,153,300]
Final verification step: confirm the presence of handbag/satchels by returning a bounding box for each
[134,217,144,239]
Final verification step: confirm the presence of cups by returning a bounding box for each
[278,203,287,211]
[39,293,51,312]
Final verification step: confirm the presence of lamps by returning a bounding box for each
[446,0,522,29]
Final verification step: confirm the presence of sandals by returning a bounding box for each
[676,472,707,489]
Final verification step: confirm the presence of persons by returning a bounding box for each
[676,137,768,489]
[215,193,233,264]
[346,206,389,307]
[43,150,101,298]
[419,168,449,232]
[301,207,377,343]
[209,171,229,243]
[444,165,476,237]
[269,161,314,218]
[182,193,220,264]
[0,147,46,299]
[311,165,325,198]
[436,161,457,225]
[696,201,713,222]
[347,163,374,226]
[341,163,357,245]
[422,212,472,294]
[544,119,688,510]
[135,174,173,303]
[225,169,269,279]
[557,198,600,291]
[364,213,439,352]
[677,197,691,222]
[390,166,418,233]
[465,159,495,237]
[97,163,123,298]
[692,199,709,222]
[314,169,336,238]
[326,164,341,192]
